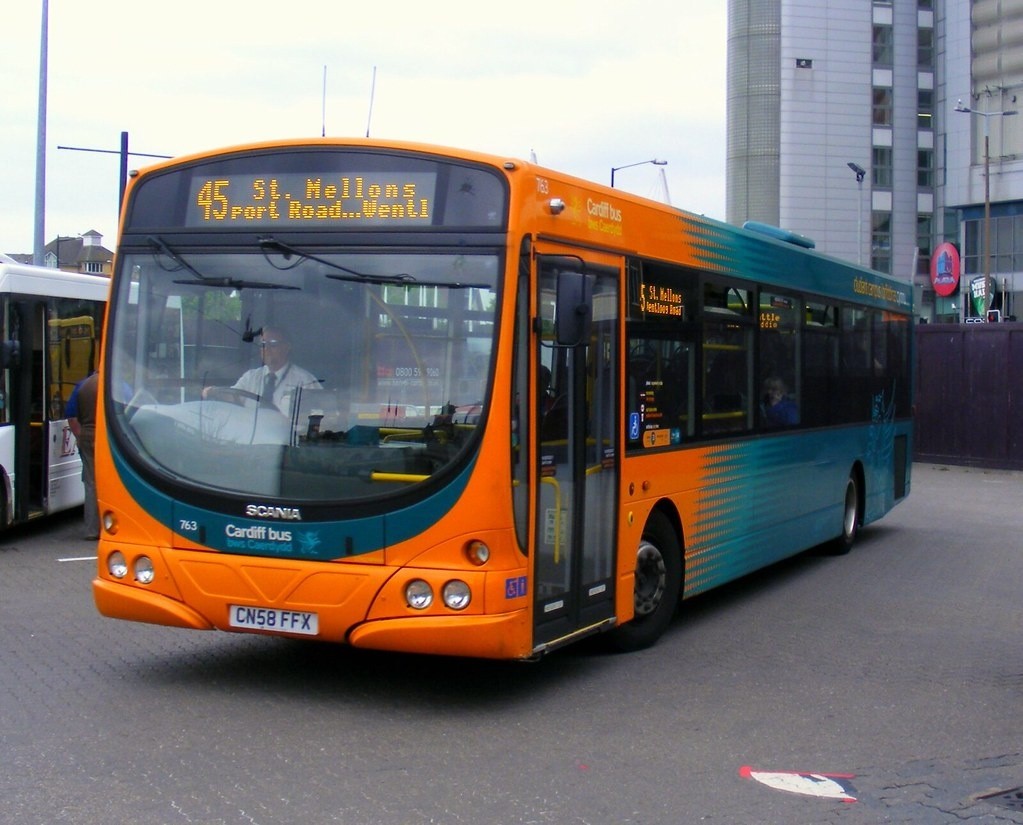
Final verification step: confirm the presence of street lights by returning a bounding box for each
[847,161,867,265]
[610,158,668,189]
[955,106,1018,323]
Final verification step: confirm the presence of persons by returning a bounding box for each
[66,352,133,539]
[521,322,884,443]
[200,325,339,432]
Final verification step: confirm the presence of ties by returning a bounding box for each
[260,375,276,409]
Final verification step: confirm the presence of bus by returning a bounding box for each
[0,262,180,537]
[91,138,916,658]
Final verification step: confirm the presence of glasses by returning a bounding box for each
[257,339,284,348]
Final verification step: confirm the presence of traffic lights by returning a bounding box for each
[987,310,1000,324]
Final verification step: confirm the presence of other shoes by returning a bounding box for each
[86,535,98,540]
